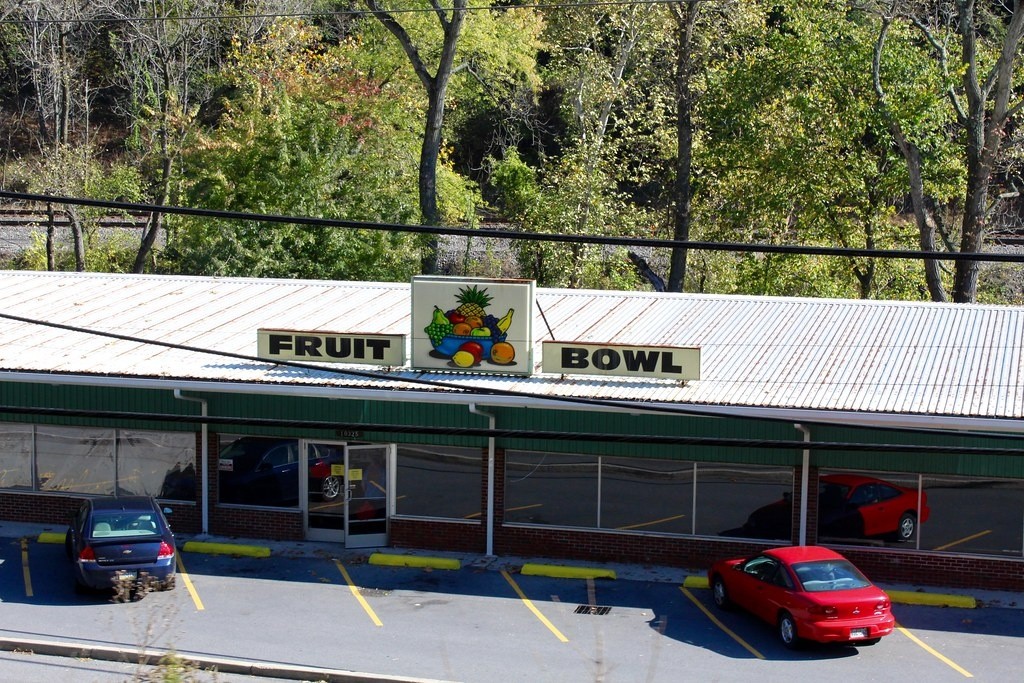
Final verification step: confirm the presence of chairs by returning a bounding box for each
[93,522,111,531]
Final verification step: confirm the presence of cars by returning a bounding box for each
[222,436,348,502]
[65,494,178,597]
[708,545,895,647]
[777,473,929,541]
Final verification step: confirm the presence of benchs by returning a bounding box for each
[802,578,855,591]
